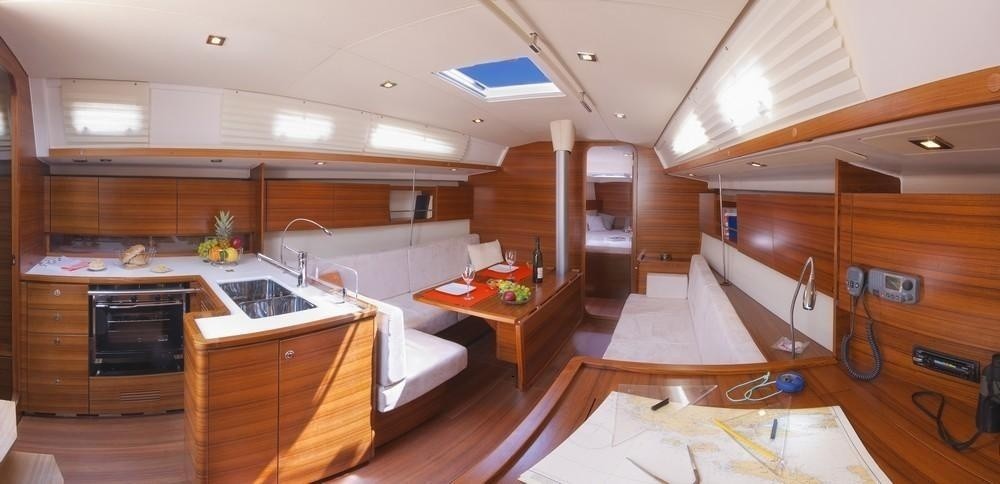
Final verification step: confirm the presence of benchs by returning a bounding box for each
[602,252,767,367]
[362,292,477,453]
[313,232,486,347]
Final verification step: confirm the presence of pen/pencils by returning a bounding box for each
[714,419,784,464]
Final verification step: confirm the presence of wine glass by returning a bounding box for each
[462,264,475,299]
[505,248,516,279]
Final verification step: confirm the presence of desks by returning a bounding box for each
[414,260,582,392]
[459,342,1000,482]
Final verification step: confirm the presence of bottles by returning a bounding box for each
[975,354,1000,433]
[532,236,544,283]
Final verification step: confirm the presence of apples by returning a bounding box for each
[504,292,517,301]
[231,237,244,249]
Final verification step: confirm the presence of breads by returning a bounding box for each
[119,242,147,270]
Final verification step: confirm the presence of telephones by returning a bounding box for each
[976,354,1000,433]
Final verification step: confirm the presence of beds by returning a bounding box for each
[585,211,632,297]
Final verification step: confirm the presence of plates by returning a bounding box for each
[487,262,520,273]
[435,282,477,294]
[150,267,172,272]
[87,266,106,271]
[498,292,535,304]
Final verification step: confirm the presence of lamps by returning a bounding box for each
[789,253,817,362]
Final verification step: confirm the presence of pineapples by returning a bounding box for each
[217,239,230,248]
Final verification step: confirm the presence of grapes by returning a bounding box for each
[197,238,218,259]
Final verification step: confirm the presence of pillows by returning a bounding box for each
[465,240,505,271]
[600,212,617,231]
[589,213,605,233]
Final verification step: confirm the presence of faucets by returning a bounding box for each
[257,252,307,288]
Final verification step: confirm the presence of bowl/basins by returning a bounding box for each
[208,247,243,268]
[114,245,160,267]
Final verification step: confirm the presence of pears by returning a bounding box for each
[224,247,238,262]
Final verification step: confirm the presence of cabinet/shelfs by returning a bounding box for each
[177,174,261,237]
[267,175,391,230]
[636,249,691,294]
[95,174,178,239]
[26,280,90,413]
[277,314,375,483]
[50,174,99,235]
[422,186,471,220]
[89,372,186,414]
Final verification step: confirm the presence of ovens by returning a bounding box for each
[87,283,197,376]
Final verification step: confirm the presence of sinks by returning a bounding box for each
[218,280,291,301]
[238,297,315,318]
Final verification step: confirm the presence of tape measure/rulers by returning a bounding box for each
[776,372,804,393]
[611,383,718,448]
[713,395,792,477]
